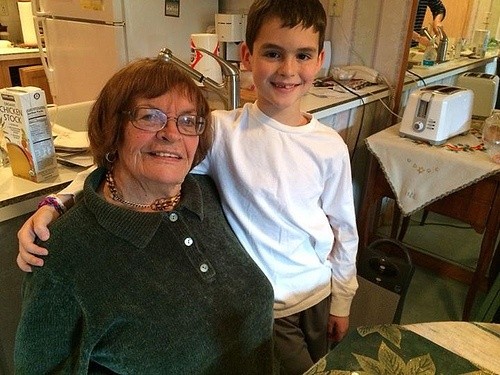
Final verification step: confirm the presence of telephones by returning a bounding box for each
[338,65,378,83]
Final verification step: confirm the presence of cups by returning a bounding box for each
[482,113,500,164]
[332,65,356,93]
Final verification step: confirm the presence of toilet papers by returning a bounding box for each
[191,34,222,86]
[474,30,489,54]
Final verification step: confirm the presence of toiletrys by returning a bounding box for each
[455,39,461,58]
[422,38,437,66]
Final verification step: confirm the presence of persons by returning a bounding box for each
[15,57,274,375]
[17,0,359,375]
[410,0,446,48]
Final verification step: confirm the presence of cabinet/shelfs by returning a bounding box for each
[0,52,53,105]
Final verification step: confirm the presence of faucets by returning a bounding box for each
[423,26,448,62]
[158,48,240,111]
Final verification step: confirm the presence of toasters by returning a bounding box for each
[454,72,500,120]
[398,83,474,146]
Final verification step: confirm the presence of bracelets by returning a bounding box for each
[38,194,66,215]
[415,34,420,42]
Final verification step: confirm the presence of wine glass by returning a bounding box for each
[47,103,58,128]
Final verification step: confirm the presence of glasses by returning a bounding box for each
[120,100,206,137]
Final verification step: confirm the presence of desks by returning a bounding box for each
[356,115,500,323]
[0,75,392,374]
[302,322,500,375]
[400,48,500,117]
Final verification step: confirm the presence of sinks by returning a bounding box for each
[407,51,468,69]
[48,100,97,135]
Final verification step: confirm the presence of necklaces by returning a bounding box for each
[106,171,181,211]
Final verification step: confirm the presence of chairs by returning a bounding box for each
[352,235,414,326]
